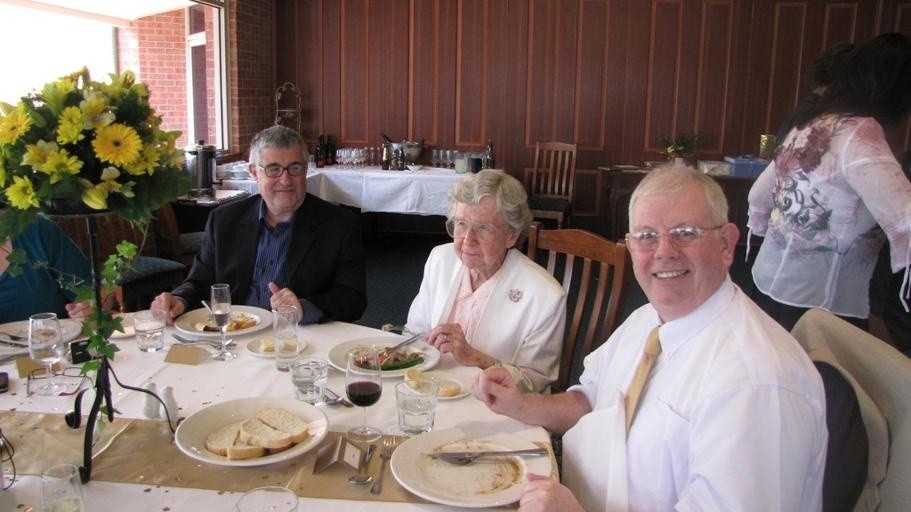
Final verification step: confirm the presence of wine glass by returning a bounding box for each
[28,311,67,396]
[346,346,383,441]
[211,284,238,360]
[43,464,87,512]
[336,147,370,171]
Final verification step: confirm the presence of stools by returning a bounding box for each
[96,255,185,312]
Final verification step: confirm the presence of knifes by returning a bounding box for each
[425,450,550,460]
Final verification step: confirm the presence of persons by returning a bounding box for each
[738,42,865,299]
[0,195,113,324]
[747,31,911,335]
[402,169,566,399]
[470,167,827,512]
[151,126,370,327]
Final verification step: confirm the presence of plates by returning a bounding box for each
[402,375,475,403]
[326,335,442,377]
[1,319,84,355]
[389,426,554,510]
[243,334,308,359]
[173,396,329,467]
[174,304,274,336]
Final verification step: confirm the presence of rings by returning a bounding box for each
[444,333,448,342]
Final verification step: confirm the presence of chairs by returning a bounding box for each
[528,140,578,229]
[788,307,911,512]
[524,222,627,394]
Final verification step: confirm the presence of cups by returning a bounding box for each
[394,378,439,433]
[236,485,300,512]
[431,149,487,173]
[132,309,167,353]
[272,305,299,373]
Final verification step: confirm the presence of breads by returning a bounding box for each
[404,365,462,396]
[204,405,309,459]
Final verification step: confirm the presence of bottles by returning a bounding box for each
[397,147,405,170]
[141,381,160,419]
[382,145,389,170]
[316,135,327,167]
[326,136,335,164]
[487,139,495,169]
[390,150,398,169]
[159,386,178,421]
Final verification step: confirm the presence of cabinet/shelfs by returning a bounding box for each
[176,189,251,232]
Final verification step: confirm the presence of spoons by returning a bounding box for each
[320,394,347,405]
[349,442,375,484]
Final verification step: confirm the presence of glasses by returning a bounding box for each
[629,224,728,248]
[255,162,307,178]
[448,220,511,238]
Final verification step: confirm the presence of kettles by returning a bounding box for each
[184,140,222,202]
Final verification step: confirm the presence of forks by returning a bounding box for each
[370,438,396,495]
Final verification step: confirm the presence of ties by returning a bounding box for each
[625,329,662,434]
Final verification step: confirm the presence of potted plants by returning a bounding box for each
[658,129,702,166]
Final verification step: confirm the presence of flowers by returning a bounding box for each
[0,65,186,214]
